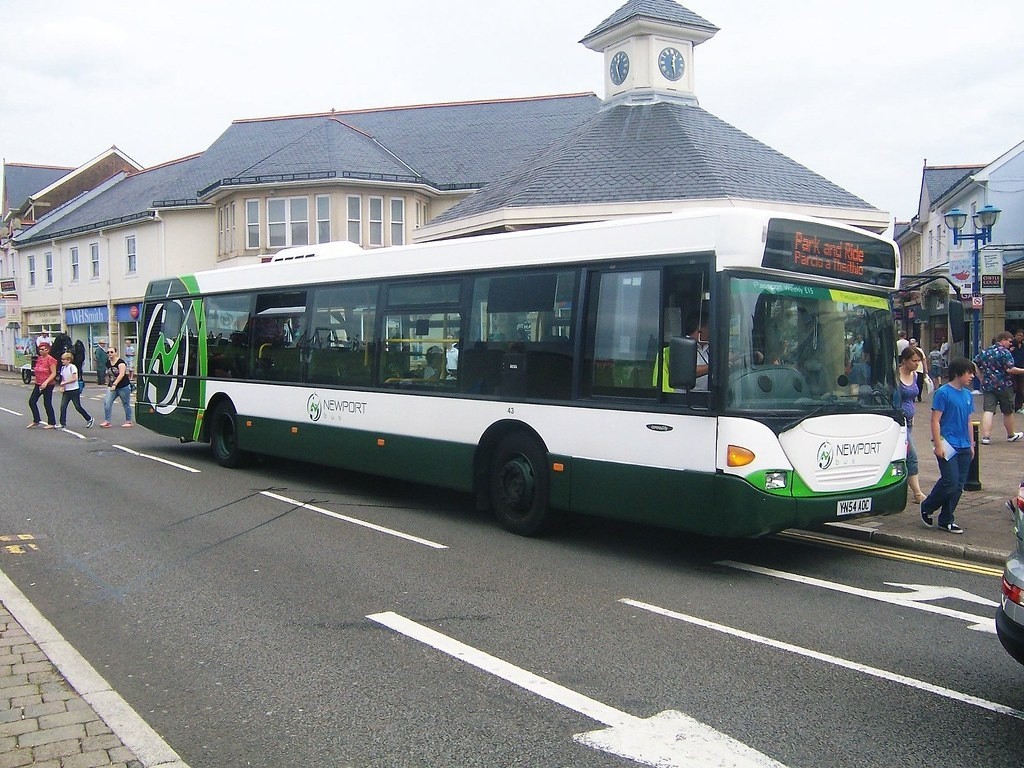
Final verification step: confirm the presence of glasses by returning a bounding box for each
[40,349,45,351]
[1006,339,1013,343]
[108,351,113,354]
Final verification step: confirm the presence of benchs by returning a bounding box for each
[216,340,411,389]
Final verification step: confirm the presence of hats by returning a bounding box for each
[99,339,107,344]
[41,329,49,333]
[427,345,443,354]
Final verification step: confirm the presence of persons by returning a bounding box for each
[26,342,56,429]
[681,311,763,395]
[894,347,927,504]
[99,346,133,427]
[835,330,1024,444]
[36,329,52,347]
[424,332,461,381]
[94,339,109,386]
[74,340,85,381]
[125,341,135,379]
[921,358,976,533]
[55,353,95,430]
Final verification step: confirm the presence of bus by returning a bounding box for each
[135,196,969,567]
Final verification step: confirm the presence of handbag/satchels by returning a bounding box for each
[130,383,136,393]
[924,374,935,394]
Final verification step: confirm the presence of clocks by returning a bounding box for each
[609,51,630,85]
[658,48,686,81]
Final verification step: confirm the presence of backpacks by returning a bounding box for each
[61,365,85,394]
[930,352,942,369]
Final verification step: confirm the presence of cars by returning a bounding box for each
[994,481,1024,667]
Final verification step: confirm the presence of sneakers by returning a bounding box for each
[938,519,964,534]
[86,417,95,428]
[981,437,990,445]
[1006,499,1017,522]
[919,502,934,528]
[55,424,66,428]
[99,420,112,428]
[122,420,133,427]
[1007,432,1023,442]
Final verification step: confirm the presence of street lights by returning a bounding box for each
[940,201,1001,394]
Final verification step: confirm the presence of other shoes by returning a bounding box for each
[1016,407,1024,414]
[913,493,928,503]
[26,422,39,428]
[42,425,55,429]
